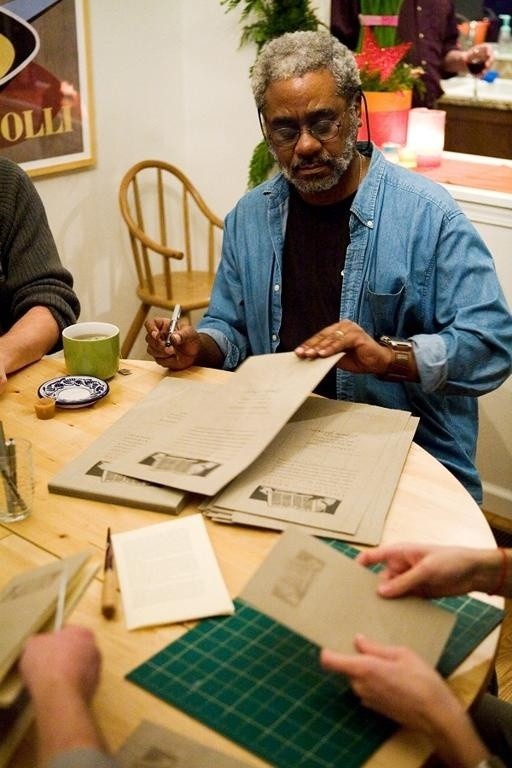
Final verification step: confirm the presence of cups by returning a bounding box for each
[410,109,446,167]
[1,436,34,522]
[62,321,120,379]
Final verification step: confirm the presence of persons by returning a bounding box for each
[145,31,512,508]
[331,0,494,108]
[320,541,512,768]
[17,625,118,768]
[0,156,81,397]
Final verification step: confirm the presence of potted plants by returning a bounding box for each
[355,61,428,151]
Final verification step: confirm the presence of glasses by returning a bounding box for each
[249,106,352,149]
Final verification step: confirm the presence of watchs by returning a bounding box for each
[378,335,412,384]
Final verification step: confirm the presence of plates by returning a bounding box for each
[37,376,108,409]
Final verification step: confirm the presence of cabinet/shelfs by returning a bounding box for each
[420,150,512,542]
[429,75,511,156]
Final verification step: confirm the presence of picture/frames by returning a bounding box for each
[0,0,98,184]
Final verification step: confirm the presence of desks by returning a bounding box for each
[0,351,511,767]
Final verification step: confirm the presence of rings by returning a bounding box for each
[335,331,344,338]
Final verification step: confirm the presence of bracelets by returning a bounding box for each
[476,755,507,768]
[489,549,507,595]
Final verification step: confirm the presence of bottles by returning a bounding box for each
[383,143,398,162]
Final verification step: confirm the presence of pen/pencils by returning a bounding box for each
[0,421,28,518]
[166,305,182,346]
[101,543,116,620]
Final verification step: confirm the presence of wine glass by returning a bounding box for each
[464,46,485,102]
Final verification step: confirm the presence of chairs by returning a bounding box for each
[114,159,225,363]
